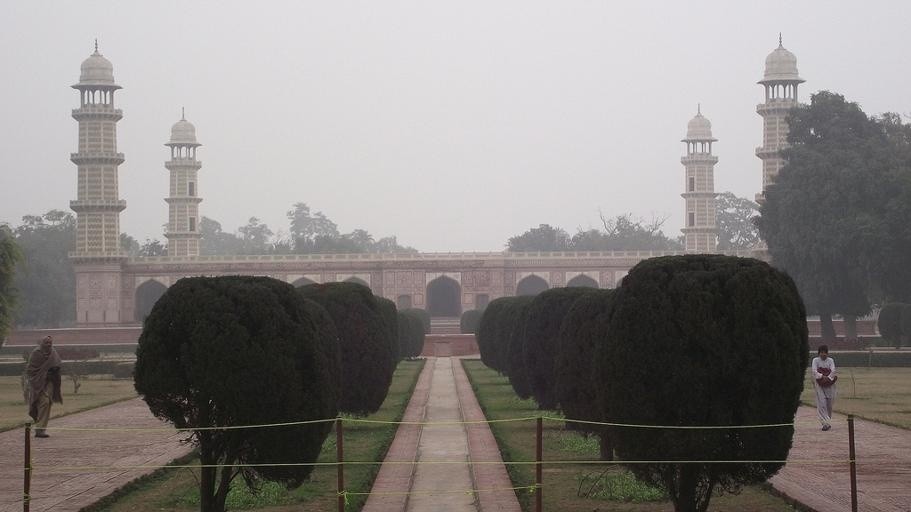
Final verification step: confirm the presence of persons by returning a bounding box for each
[812,346,836,432]
[25,335,62,438]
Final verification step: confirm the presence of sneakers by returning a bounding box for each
[822,424,831,431]
[35,433,49,437]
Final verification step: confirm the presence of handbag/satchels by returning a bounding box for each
[817,367,837,385]
